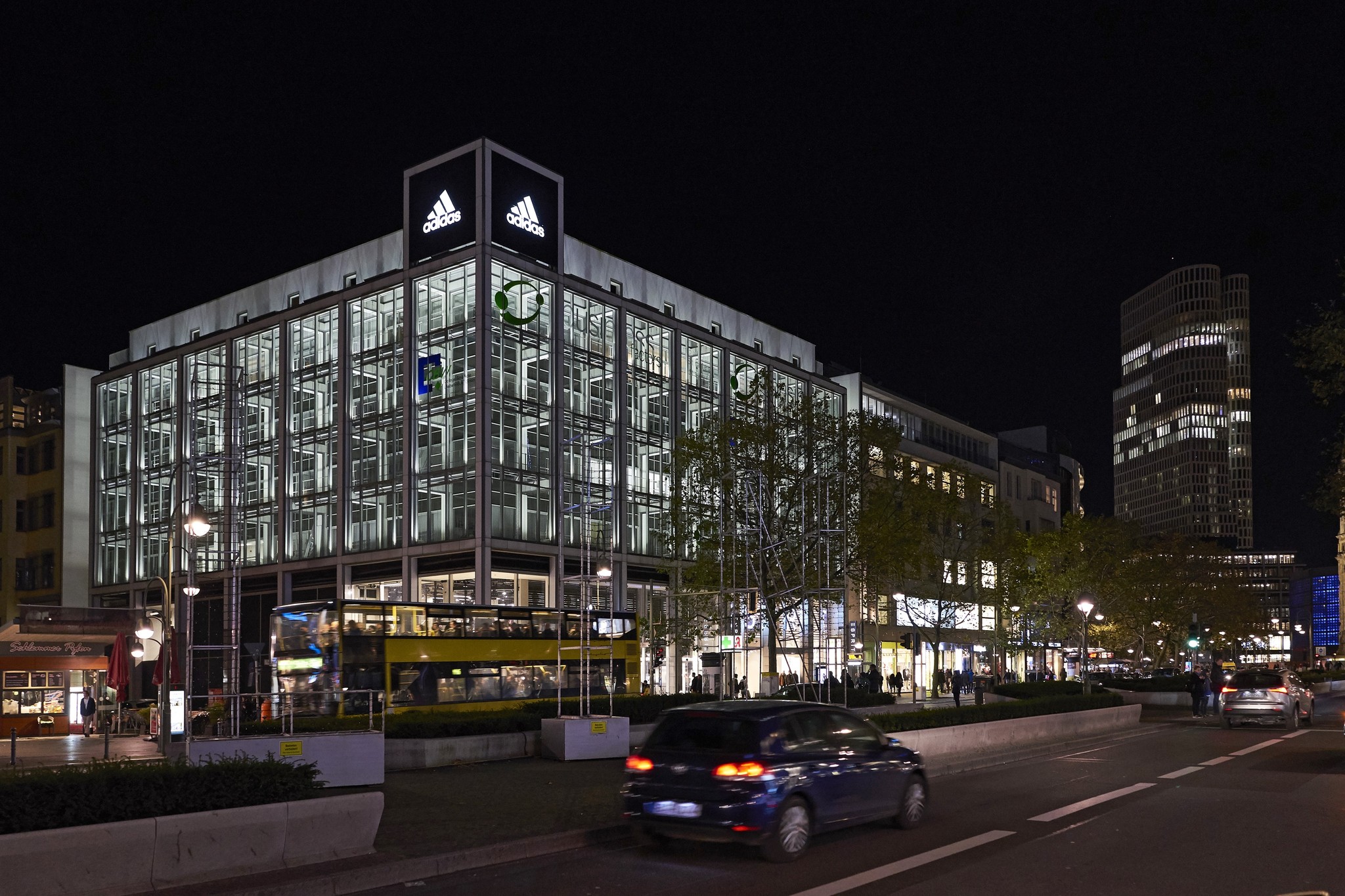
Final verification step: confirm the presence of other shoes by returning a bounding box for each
[1192,713,1213,718]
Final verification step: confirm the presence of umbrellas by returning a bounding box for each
[152,626,180,690]
[106,632,131,734]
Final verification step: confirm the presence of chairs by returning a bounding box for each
[111,715,130,734]
[344,627,576,636]
[37,715,55,737]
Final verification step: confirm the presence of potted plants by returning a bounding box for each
[205,702,226,736]
[136,703,158,735]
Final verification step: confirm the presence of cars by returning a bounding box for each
[771,682,823,696]
[107,699,158,726]
[621,699,930,863]
[1082,666,1186,686]
[1219,668,1315,731]
[1221,661,1267,681]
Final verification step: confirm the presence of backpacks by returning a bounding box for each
[888,679,893,685]
[738,682,746,690]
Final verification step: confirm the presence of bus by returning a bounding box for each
[248,598,641,718]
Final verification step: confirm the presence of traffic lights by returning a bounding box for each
[900,633,910,649]
[653,645,663,667]
[1188,622,1199,649]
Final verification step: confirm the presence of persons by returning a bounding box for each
[480,620,597,638]
[284,621,340,650]
[80,691,96,737]
[981,669,1018,686]
[1187,658,1224,718]
[224,686,250,736]
[823,664,883,694]
[690,673,702,693]
[1293,661,1343,671]
[779,670,799,689]
[349,620,390,636]
[728,674,748,700]
[938,669,978,708]
[888,672,903,696]
[442,669,580,697]
[415,621,461,637]
[1079,665,1123,677]
[642,680,650,696]
[1029,666,1067,682]
[1236,663,1280,669]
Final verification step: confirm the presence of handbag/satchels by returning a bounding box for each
[899,677,903,684]
[1185,674,1195,693]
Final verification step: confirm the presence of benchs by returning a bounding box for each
[438,687,494,701]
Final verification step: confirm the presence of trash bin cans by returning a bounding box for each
[973,674,994,704]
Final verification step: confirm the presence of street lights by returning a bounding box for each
[1294,608,1314,670]
[1127,615,1162,674]
[131,461,211,752]
[1066,592,1104,682]
[1170,612,1294,672]
[854,564,905,695]
[973,576,1020,686]
